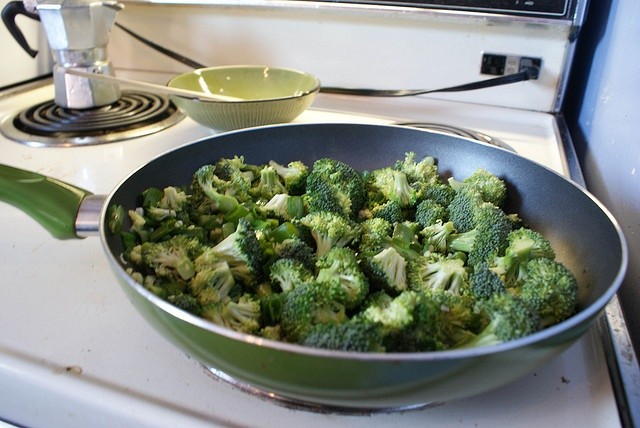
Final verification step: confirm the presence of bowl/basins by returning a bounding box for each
[168,66,321,133]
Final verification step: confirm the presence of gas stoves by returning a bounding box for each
[1,0,637,426]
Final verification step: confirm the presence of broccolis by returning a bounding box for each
[125,150,578,351]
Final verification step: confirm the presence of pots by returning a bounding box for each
[0,121,629,411]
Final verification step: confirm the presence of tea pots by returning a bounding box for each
[1,0,128,109]
